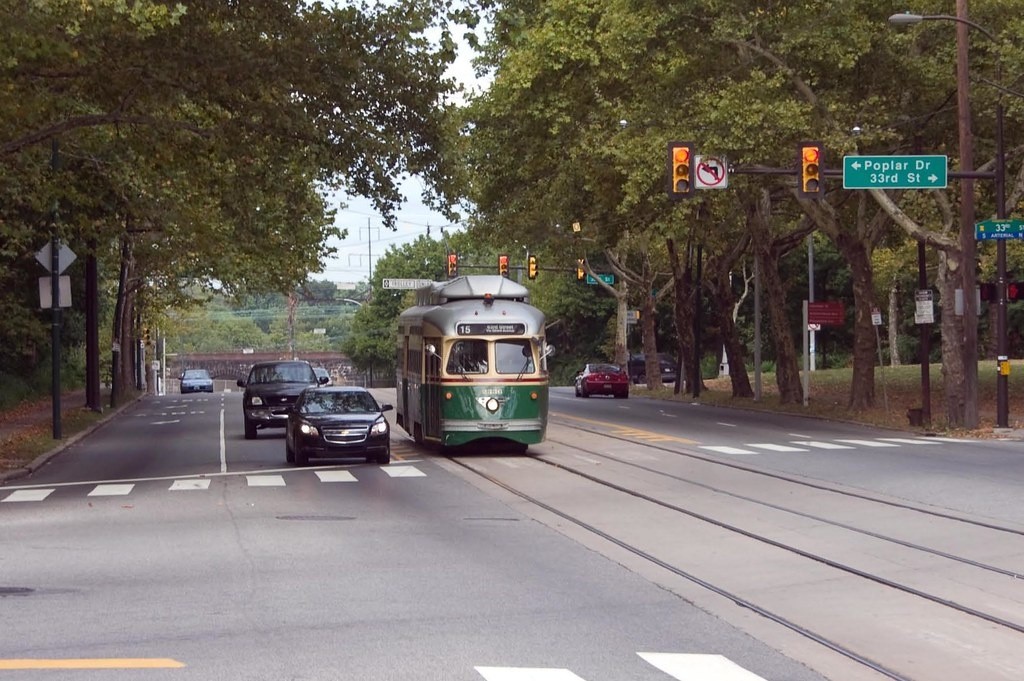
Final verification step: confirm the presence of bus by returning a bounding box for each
[398,276,548,455]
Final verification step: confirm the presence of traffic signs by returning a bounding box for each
[975,220,1024,239]
[844,155,947,189]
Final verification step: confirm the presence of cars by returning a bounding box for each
[286,387,394,467]
[313,368,334,388]
[630,352,681,383]
[180,369,213,393]
[574,364,629,398]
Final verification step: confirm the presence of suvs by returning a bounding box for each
[237,360,329,439]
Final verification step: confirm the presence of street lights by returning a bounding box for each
[891,11,1011,428]
[336,298,374,388]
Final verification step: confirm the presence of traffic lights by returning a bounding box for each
[981,283,1024,301]
[448,255,457,277]
[673,147,690,193]
[529,256,538,279]
[577,259,584,280]
[802,147,820,194]
[499,255,509,277]
